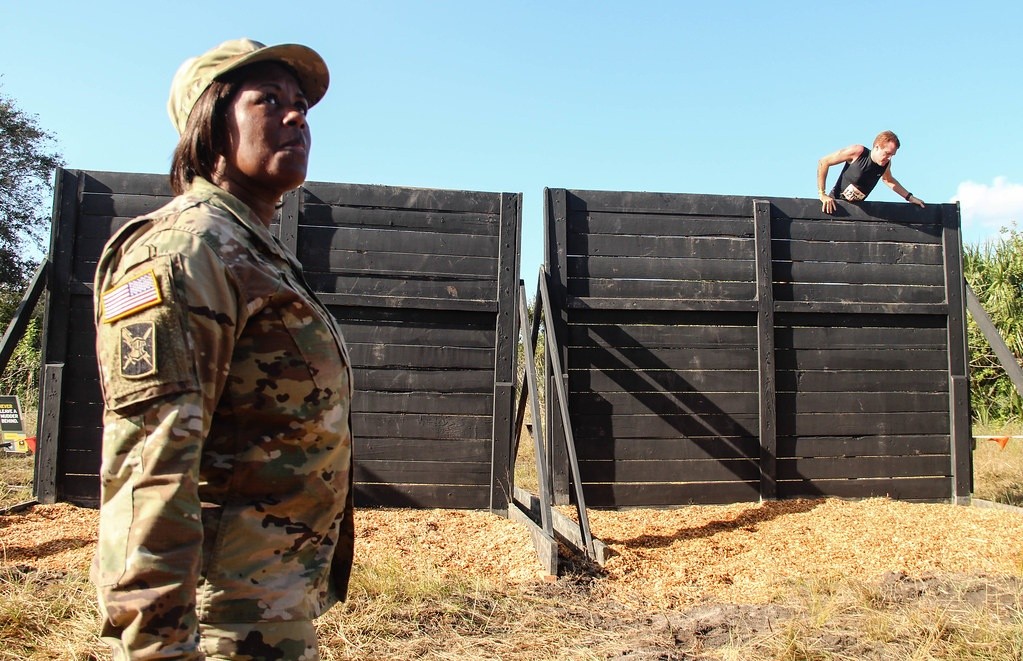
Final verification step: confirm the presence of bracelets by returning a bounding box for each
[906,193,912,201]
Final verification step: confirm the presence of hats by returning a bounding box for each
[164,40,327,140]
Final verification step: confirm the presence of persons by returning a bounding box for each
[90,37,355,660]
[818,130,925,213]
